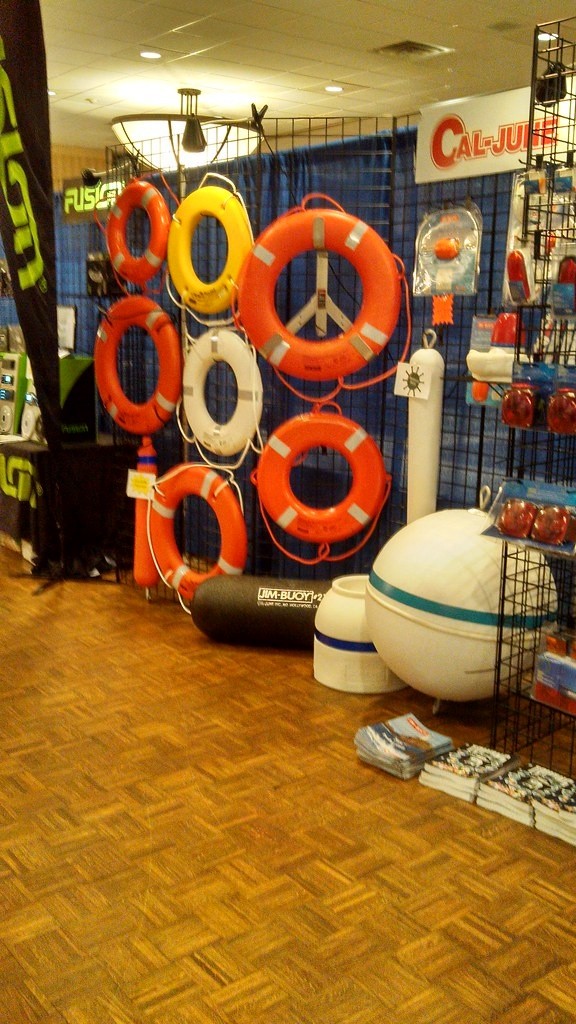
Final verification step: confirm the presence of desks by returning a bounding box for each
[0,431,142,572]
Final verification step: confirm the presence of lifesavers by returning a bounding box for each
[144,461,247,603]
[179,329,264,456]
[94,292,183,434]
[165,186,253,314]
[106,178,170,284]
[257,412,384,541]
[245,208,403,381]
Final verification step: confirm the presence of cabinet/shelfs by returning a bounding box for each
[481,11,576,782]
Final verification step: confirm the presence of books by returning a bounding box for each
[352,711,454,782]
[415,742,521,802]
[531,781,576,847]
[477,758,575,828]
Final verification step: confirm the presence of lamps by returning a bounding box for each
[111,87,264,174]
